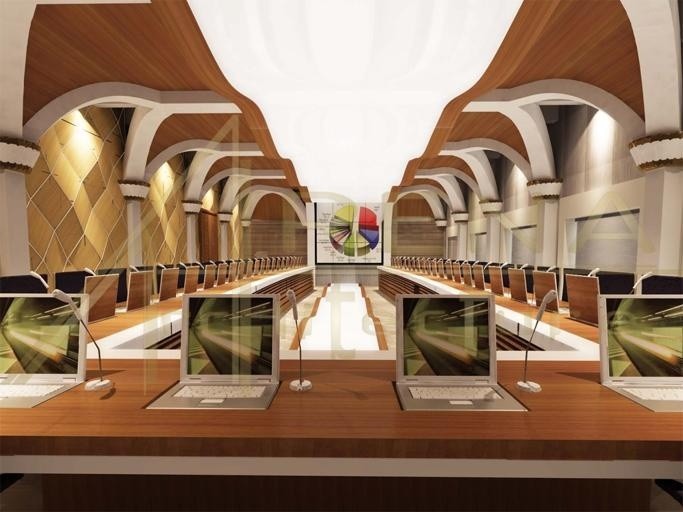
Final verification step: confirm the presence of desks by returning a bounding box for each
[0,264,683,512]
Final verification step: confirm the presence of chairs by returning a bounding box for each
[1,260,235,305]
[466,260,683,302]
